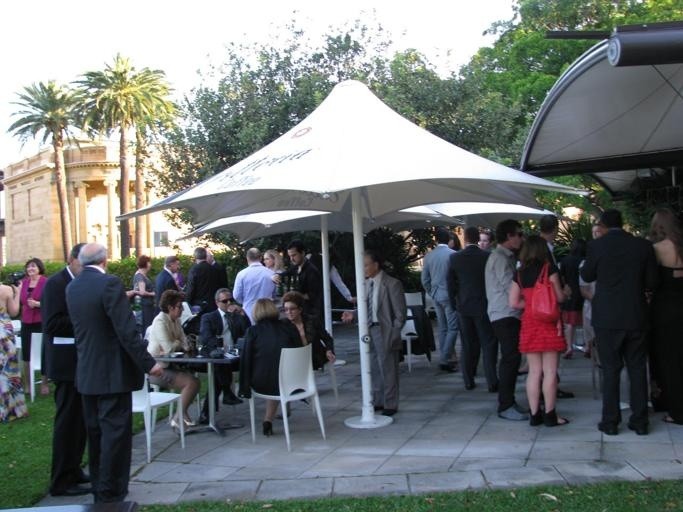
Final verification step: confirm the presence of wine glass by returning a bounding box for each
[171,333,239,359]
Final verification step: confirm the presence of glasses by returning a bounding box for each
[218,297,235,304]
[513,230,524,237]
[175,304,182,309]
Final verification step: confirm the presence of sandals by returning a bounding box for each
[542,407,570,427]
[528,407,543,426]
[661,414,683,425]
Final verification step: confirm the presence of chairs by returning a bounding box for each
[401,292,430,373]
[30,333,41,403]
[132,302,339,464]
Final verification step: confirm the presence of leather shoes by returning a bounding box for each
[374,404,384,411]
[437,360,458,373]
[49,484,94,496]
[76,470,93,485]
[199,407,209,425]
[488,384,498,393]
[627,420,649,436]
[464,382,476,391]
[381,409,397,416]
[598,422,618,435]
[222,392,244,405]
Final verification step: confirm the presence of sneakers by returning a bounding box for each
[513,401,531,413]
[556,388,575,399]
[515,369,529,376]
[498,406,529,421]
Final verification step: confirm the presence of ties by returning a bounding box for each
[367,280,374,330]
[224,312,235,341]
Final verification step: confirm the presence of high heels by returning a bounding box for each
[560,349,574,360]
[170,418,188,433]
[183,417,197,426]
[263,421,272,437]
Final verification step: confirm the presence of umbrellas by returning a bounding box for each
[175,199,560,368]
[112,77,584,425]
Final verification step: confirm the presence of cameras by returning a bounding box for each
[8,271,25,284]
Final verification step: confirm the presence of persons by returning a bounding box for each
[200,239,406,433]
[421,210,682,435]
[1,209,228,503]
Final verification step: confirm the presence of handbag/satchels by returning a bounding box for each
[182,304,210,336]
[529,260,564,325]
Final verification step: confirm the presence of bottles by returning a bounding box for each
[274,275,299,295]
[132,281,141,311]
[179,284,187,293]
